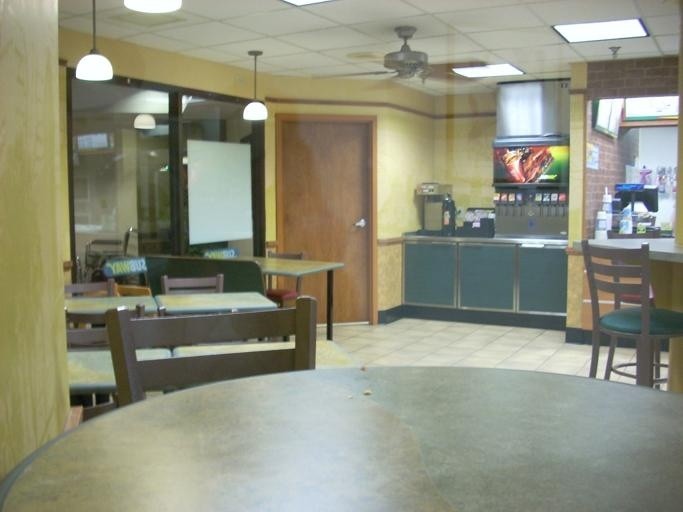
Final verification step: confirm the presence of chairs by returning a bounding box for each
[161,273,224,295]
[581,239,682,388]
[65,279,115,296]
[268,250,304,295]
[144,257,266,296]
[107,297,318,406]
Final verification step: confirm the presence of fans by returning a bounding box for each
[314,28,486,90]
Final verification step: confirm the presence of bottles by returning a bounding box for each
[600,187,612,232]
[593,211,606,240]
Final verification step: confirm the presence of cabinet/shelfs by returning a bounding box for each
[402,231,568,325]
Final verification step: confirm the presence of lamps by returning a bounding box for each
[130,114,156,132]
[76,0,114,81]
[243,50,269,122]
